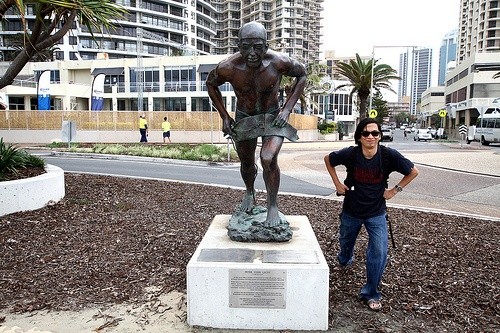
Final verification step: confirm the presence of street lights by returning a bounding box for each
[370,62,372,108]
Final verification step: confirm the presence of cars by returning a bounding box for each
[404,127,411,133]
[414,129,432,141]
[435,130,447,140]
[400,126,405,130]
[381,130,393,141]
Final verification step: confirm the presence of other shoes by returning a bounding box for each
[333,263,349,272]
[367,298,382,310]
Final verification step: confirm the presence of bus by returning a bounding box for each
[475,114,500,145]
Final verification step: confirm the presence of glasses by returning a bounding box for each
[361,130,380,136]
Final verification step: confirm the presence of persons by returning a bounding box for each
[138,115,149,142]
[206,22,308,228]
[162,117,171,143]
[403,129,408,140]
[324,118,418,310]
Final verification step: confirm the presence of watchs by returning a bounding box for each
[395,185,403,192]
